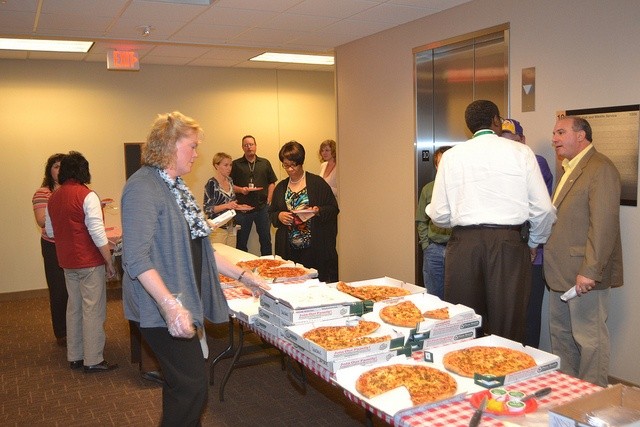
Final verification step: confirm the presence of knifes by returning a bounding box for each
[469,395,487,427]
[520,387,552,402]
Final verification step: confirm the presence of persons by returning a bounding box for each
[268,140,339,283]
[32,153,68,347]
[45,151,116,372]
[229,135,278,256]
[425,99,557,349]
[203,152,256,248]
[501,120,553,349]
[415,146,453,300]
[543,116,623,387]
[121,111,230,427]
[318,139,337,200]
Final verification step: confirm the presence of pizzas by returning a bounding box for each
[303,319,393,350]
[423,306,451,319]
[259,266,306,278]
[380,300,424,328]
[444,345,539,379]
[337,281,411,303]
[238,257,286,267]
[356,362,456,406]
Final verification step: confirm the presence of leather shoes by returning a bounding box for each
[70,360,83,370]
[84,359,118,374]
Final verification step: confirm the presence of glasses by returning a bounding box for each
[242,143,255,147]
[282,162,299,169]
[496,114,505,123]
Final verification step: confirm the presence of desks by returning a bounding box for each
[220,312,609,426]
[210,240,318,383]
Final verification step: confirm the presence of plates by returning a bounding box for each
[471,390,538,416]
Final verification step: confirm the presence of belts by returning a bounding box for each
[454,224,522,233]
[429,239,448,246]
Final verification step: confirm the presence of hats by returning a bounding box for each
[502,119,523,135]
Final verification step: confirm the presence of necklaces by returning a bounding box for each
[290,171,306,183]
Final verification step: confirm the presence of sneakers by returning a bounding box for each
[57,337,67,347]
[142,371,164,385]
